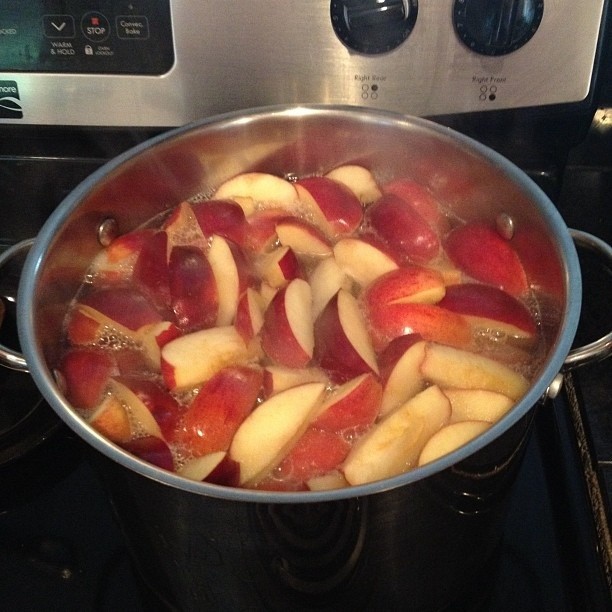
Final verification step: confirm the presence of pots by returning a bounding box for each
[0,104,611,503]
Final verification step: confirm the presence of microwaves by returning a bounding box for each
[1,0,611,612]
